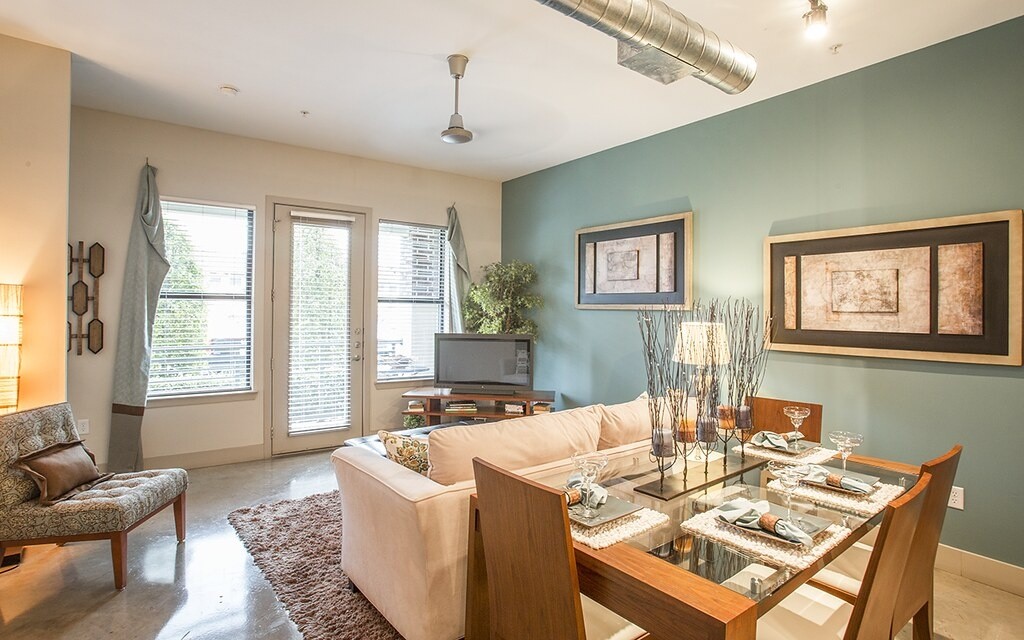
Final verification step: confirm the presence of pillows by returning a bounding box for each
[377,430,429,477]
[12,438,115,506]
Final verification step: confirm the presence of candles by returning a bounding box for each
[652,406,753,456]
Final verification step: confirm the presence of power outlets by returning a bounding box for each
[78,419,89,435]
[948,486,965,511]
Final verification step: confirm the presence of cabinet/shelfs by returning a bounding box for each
[402,387,557,427]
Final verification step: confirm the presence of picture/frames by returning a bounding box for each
[762,209,1023,367]
[575,211,693,311]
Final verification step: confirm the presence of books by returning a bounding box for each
[445,400,477,414]
[408,400,424,412]
[533,403,550,414]
[504,404,525,415]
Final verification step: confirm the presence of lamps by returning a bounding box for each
[802,0,828,38]
[0,283,24,569]
[672,322,731,418]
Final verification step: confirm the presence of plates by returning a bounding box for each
[800,464,880,495]
[712,500,834,546]
[749,438,818,455]
[568,494,644,527]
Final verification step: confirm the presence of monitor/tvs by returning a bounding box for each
[433,332,534,395]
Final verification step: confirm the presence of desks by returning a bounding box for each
[465,431,921,640]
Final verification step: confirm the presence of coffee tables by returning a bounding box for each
[344,420,493,456]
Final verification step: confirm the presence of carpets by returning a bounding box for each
[228,490,406,640]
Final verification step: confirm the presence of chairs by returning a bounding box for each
[720,443,964,640]
[0,402,190,592]
[746,397,823,445]
[472,458,653,639]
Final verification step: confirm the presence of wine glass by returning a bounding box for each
[767,458,811,529]
[782,405,810,450]
[829,430,864,483]
[570,451,610,520]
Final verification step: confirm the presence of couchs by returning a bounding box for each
[331,394,701,640]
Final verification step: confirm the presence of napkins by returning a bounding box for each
[565,474,608,510]
[714,429,874,548]
